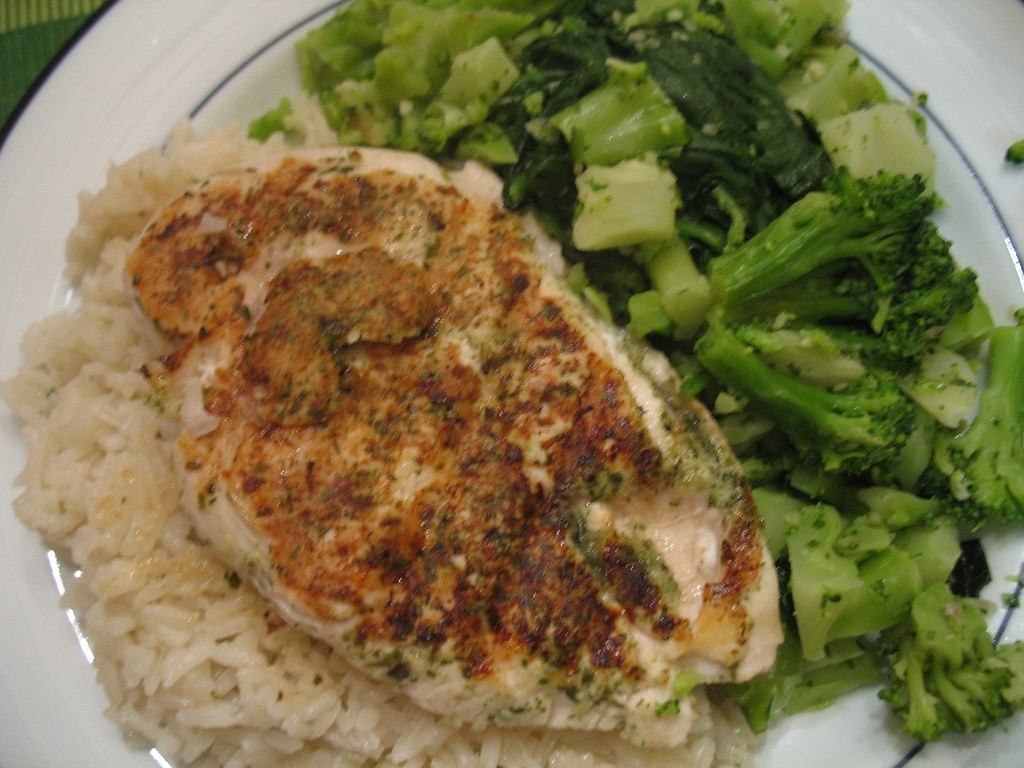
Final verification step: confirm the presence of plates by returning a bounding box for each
[0,0,1024,768]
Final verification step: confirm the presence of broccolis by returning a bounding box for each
[250,0,1024,743]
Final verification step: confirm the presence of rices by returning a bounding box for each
[0,119,766,768]
